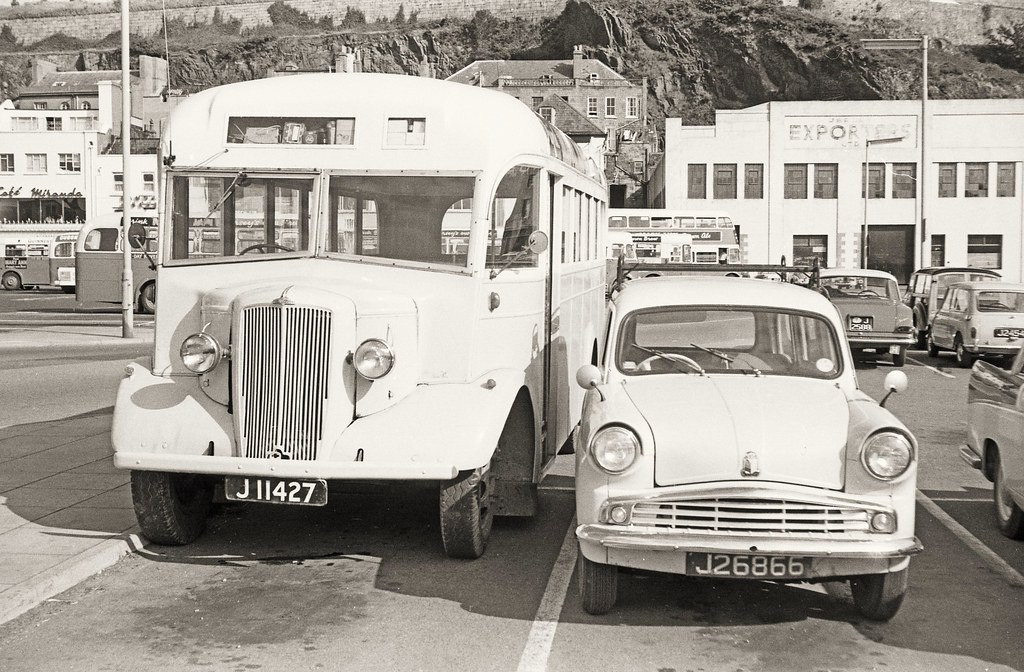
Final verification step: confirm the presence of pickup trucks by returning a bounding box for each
[959,342,1024,538]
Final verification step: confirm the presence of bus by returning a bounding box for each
[75,211,356,315]
[49,233,97,293]
[0,238,60,291]
[109,69,609,560]
[607,208,744,295]
[363,227,498,259]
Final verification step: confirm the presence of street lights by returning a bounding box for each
[863,133,905,290]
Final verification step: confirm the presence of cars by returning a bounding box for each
[806,269,919,366]
[576,271,922,620]
[902,267,1003,348]
[926,282,1024,365]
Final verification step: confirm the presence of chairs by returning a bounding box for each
[731,353,792,371]
